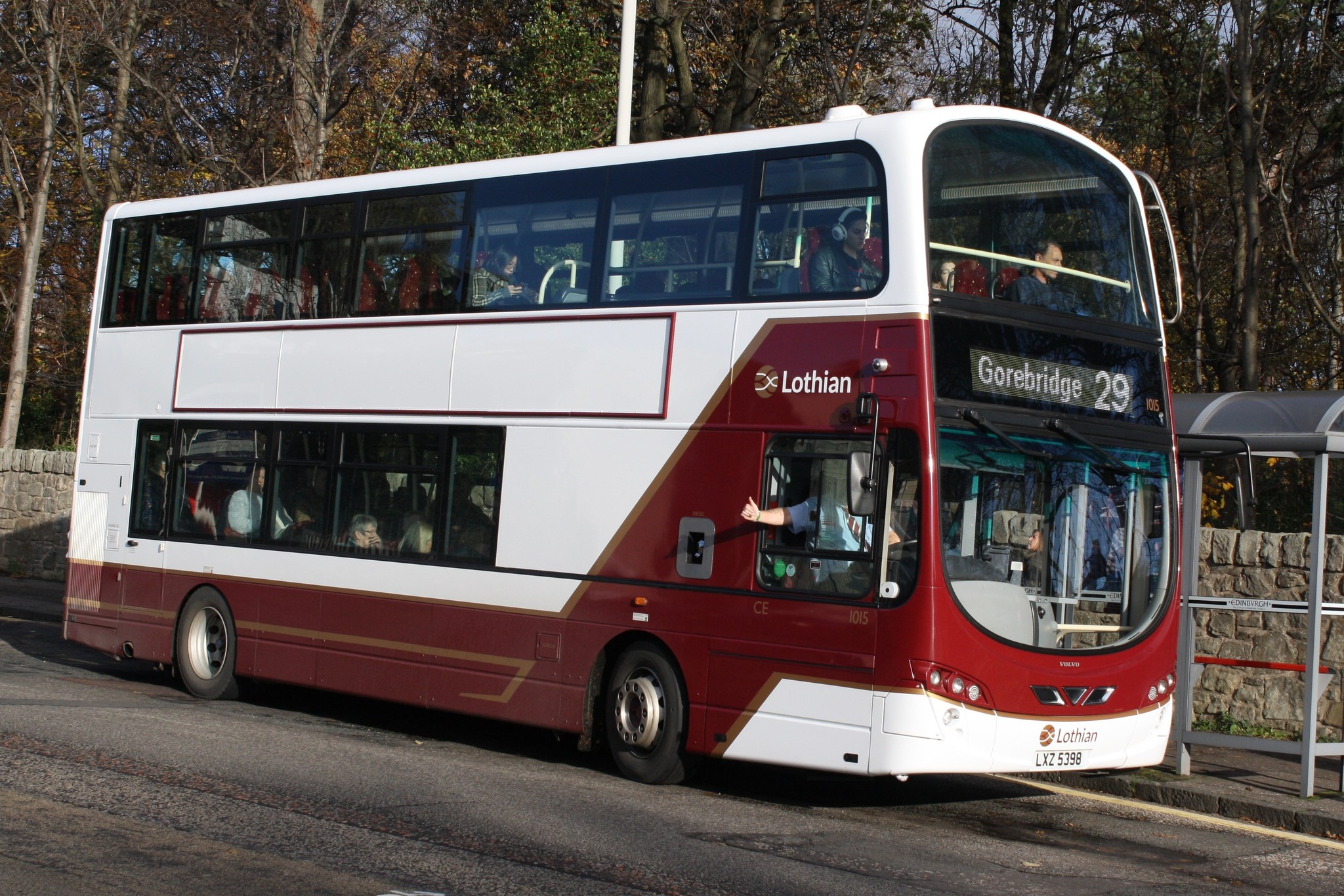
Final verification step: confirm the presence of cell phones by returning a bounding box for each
[515,283,528,291]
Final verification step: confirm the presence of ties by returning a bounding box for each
[843,508,873,552]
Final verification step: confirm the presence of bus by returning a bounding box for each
[61,96,1188,788]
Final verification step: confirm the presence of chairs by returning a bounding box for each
[140,474,405,551]
[780,475,807,549]
[114,226,1022,323]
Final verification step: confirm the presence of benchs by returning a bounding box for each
[1191,655,1336,699]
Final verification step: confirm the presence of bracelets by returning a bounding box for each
[752,510,761,522]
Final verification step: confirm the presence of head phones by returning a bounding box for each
[832,207,861,242]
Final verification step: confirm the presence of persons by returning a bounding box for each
[807,206,884,294]
[1006,237,1093,317]
[930,259,956,292]
[470,246,522,307]
[741,466,901,596]
[1022,529,1043,587]
[139,438,495,560]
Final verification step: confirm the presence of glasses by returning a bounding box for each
[361,530,378,535]
[506,263,519,272]
